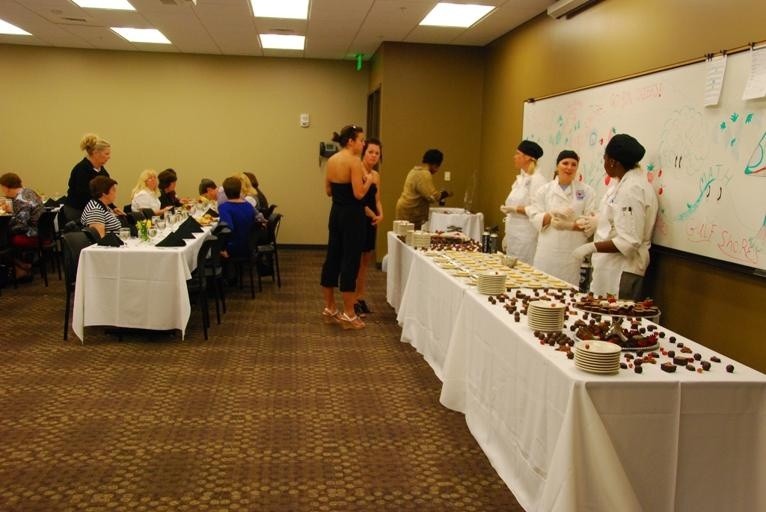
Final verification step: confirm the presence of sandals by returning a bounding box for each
[322,307,342,323]
[340,312,366,330]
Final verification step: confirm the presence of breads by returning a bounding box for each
[204,214,211,220]
[195,216,211,224]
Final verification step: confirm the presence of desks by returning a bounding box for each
[383,229,766,512]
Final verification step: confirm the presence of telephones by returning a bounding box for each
[320,142,338,158]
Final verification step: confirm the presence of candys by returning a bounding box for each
[482,289,735,373]
[397,230,483,252]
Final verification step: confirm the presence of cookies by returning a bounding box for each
[423,250,574,288]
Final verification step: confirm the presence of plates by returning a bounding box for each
[526,299,567,333]
[393,218,431,248]
[572,339,622,374]
[476,272,507,296]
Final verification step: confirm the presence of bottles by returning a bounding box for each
[464,190,474,214]
[439,198,446,206]
[481,227,499,253]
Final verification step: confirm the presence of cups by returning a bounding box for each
[5,200,13,214]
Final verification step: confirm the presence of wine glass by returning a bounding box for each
[116,204,207,248]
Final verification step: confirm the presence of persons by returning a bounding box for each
[58,135,125,255]
[77,174,122,241]
[524,150,596,288]
[395,148,454,230]
[499,139,548,267]
[0,172,52,281]
[132,168,268,282]
[571,135,658,302]
[319,123,373,329]
[353,139,384,317]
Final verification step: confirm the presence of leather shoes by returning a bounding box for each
[357,300,370,313]
[4,266,13,282]
[354,303,366,317]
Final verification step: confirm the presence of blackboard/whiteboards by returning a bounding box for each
[522,40,766,276]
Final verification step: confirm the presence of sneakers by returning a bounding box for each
[202,263,222,276]
[188,276,206,289]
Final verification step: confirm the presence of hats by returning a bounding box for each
[557,150,579,163]
[605,134,646,167]
[517,140,543,159]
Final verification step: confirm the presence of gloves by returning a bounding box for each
[500,204,517,214]
[572,242,599,260]
[549,208,598,233]
